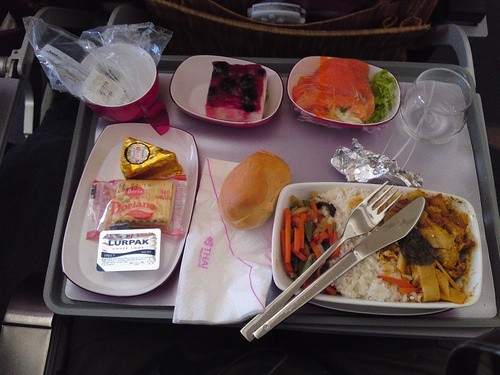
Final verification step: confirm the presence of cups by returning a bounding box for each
[79,43,170,136]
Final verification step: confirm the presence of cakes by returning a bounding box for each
[205,61,267,122]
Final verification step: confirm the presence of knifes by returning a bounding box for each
[252,196,425,339]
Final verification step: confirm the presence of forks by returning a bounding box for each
[239,180,403,341]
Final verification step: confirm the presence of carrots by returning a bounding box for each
[279,208,340,296]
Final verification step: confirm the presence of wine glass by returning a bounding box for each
[402,69,475,146]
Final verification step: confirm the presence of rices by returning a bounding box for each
[315,188,423,303]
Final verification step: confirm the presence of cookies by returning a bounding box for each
[111,180,175,227]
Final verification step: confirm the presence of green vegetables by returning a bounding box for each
[364,69,397,124]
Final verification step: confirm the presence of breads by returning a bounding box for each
[219,149,291,229]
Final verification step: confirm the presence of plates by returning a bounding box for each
[272,182,484,315]
[286,56,402,129]
[169,54,284,129]
[62,122,200,297]
